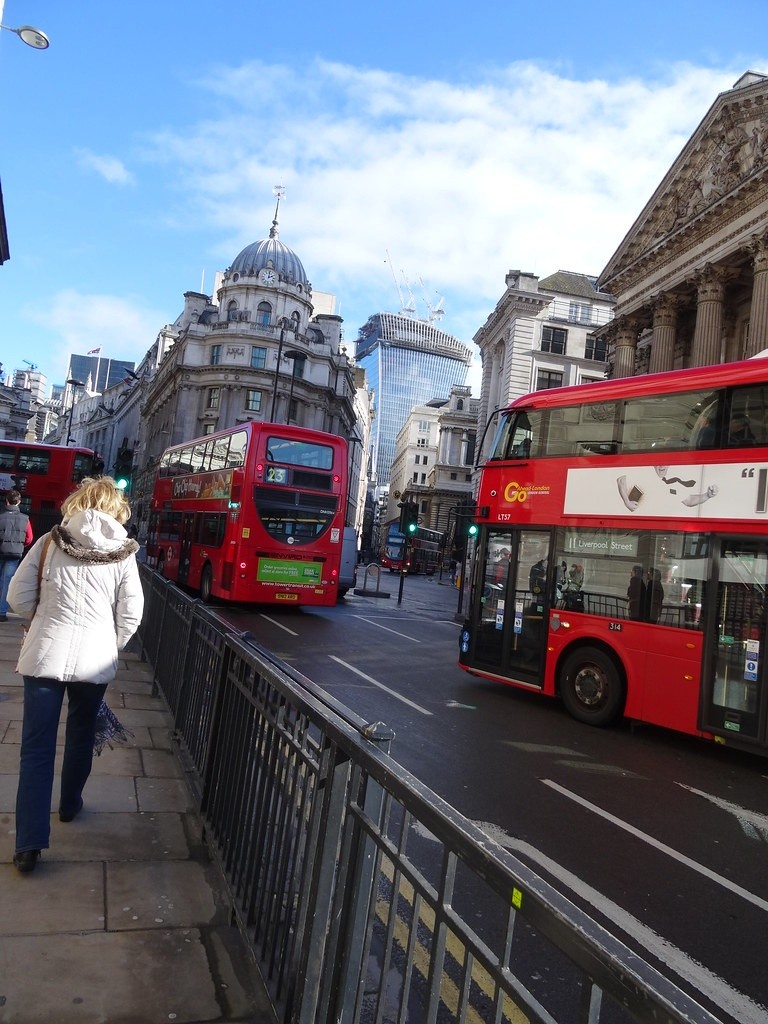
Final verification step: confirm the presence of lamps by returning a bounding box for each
[123,365,139,382]
[99,403,111,417]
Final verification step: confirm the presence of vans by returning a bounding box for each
[338,524,357,596]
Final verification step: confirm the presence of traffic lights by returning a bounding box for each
[398,502,419,535]
[113,447,135,491]
[457,500,478,537]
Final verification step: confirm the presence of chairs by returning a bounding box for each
[1,460,46,473]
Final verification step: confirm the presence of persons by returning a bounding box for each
[731,414,756,445]
[0,490,33,621]
[519,438,539,457]
[693,408,717,445]
[360,549,379,563]
[491,547,509,585]
[529,556,584,608]
[449,559,461,576]
[627,566,664,622]
[6,476,145,872]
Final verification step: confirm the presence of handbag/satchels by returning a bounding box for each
[20,623,30,656]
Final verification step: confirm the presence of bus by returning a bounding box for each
[381,522,444,575]
[456,358,768,756]
[0,439,105,539]
[142,420,348,609]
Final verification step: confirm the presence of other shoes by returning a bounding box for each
[59,796,83,822]
[0,616,8,622]
[13,847,42,872]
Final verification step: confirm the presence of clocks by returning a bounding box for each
[260,269,276,286]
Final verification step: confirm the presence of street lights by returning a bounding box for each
[65,379,85,447]
[345,438,362,526]
[283,350,308,426]
